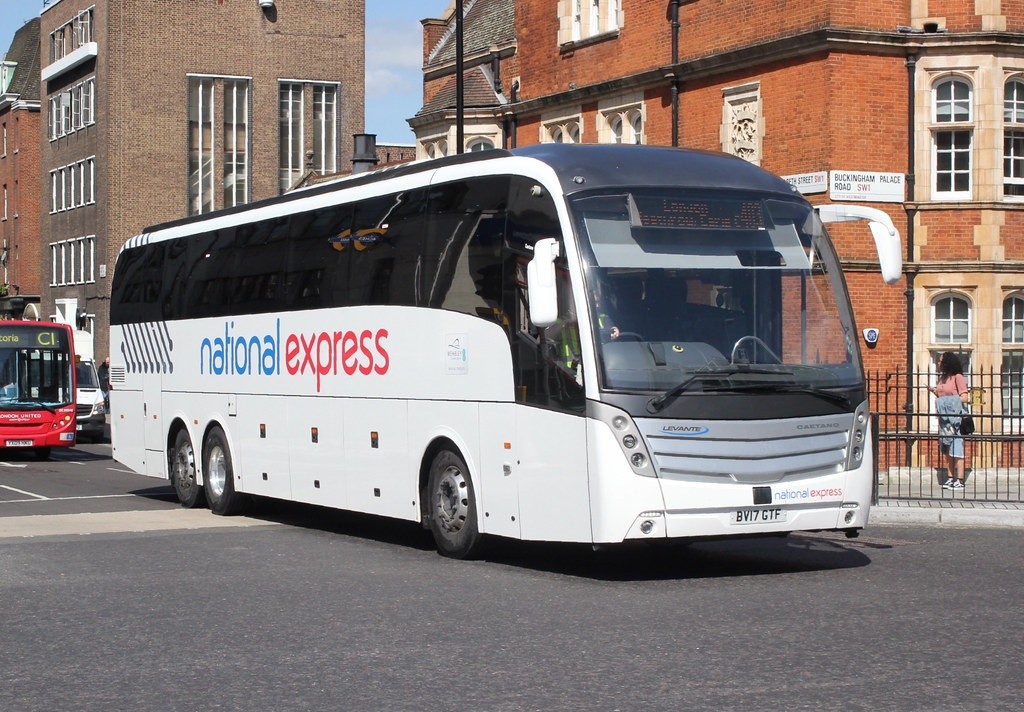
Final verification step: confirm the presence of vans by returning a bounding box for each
[72,355,106,446]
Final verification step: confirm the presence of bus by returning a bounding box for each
[0,317,77,464]
[109,141,904,562]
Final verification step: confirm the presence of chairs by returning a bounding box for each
[612,273,745,363]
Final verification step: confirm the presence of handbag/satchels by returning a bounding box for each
[961,417,974,434]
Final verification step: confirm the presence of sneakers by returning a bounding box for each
[949,478,965,491]
[942,478,954,488]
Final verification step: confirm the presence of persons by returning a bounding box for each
[97,357,110,413]
[924,349,969,490]
